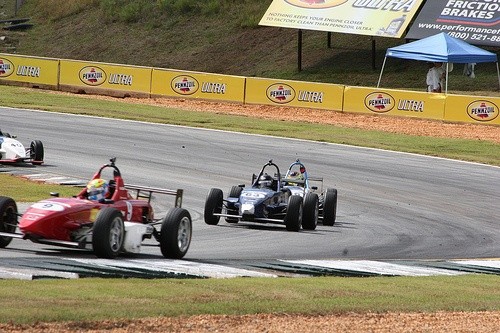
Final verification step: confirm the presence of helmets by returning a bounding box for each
[86,178,106,192]
[289,171,300,186]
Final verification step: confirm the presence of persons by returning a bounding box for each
[255,175,273,191]
[286,172,303,187]
[85,178,111,203]
[426,62,449,93]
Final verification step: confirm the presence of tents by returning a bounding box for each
[375,32,500,96]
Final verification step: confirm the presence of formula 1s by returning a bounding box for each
[204,158,338,232]
[0,129,44,166]
[0,155,192,258]
